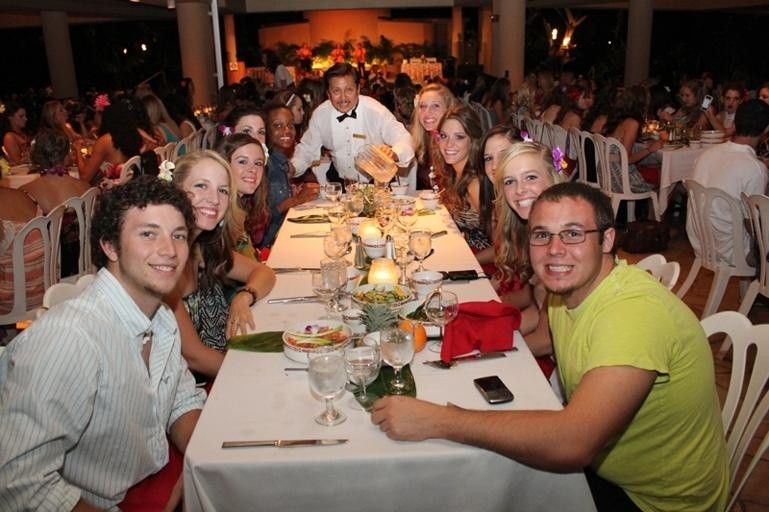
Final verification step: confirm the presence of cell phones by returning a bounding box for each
[701,94,714,112]
[473,376,514,404]
[437,269,479,280]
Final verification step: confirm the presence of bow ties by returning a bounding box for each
[337,111,356,123]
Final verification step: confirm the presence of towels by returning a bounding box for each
[440,300,521,363]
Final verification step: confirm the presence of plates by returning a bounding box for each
[350,284,412,309]
[645,120,725,149]
[418,321,446,338]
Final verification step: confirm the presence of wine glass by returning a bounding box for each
[343,337,381,410]
[425,289,459,354]
[315,173,436,320]
[381,318,414,395]
[307,345,347,426]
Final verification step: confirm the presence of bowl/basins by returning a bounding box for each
[284,321,353,366]
[343,311,365,326]
[413,271,444,294]
[419,191,440,209]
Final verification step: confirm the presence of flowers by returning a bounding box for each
[432,129,440,144]
[219,124,235,137]
[94,94,110,111]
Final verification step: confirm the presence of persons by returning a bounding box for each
[1,176,209,511]
[164,149,279,400]
[369,180,731,511]
[0,32,769,342]
[490,140,574,382]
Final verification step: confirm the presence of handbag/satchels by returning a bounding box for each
[619,219,671,253]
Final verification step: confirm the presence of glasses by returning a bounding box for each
[530,227,603,246]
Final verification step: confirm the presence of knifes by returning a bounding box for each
[423,352,508,367]
[220,437,348,448]
[271,266,320,273]
[267,295,317,302]
